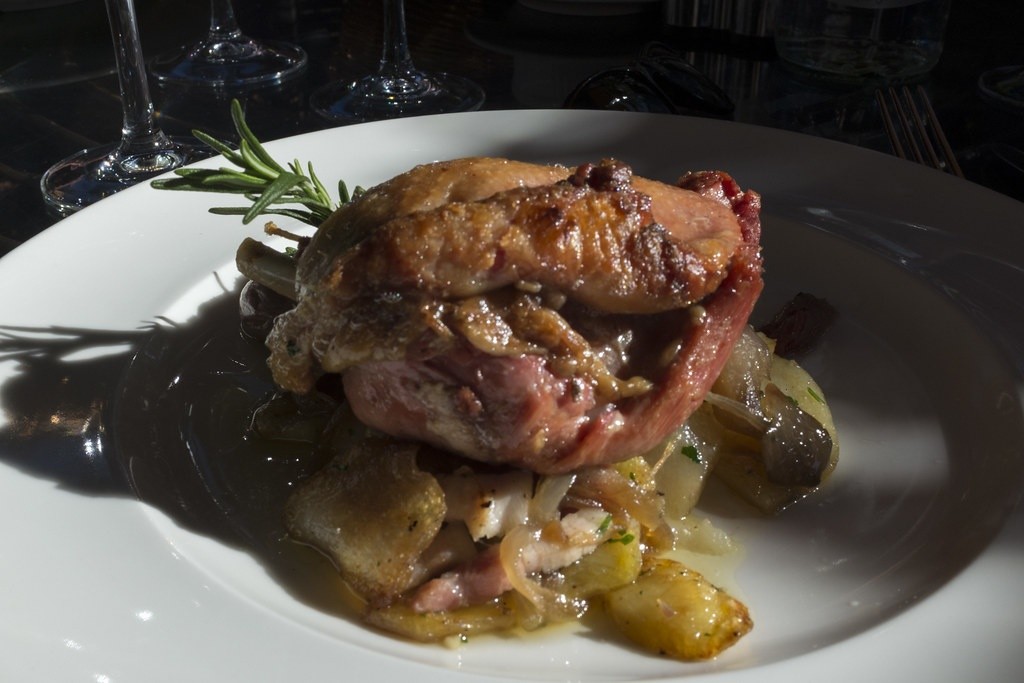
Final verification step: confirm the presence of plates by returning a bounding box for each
[0,106,1024,682]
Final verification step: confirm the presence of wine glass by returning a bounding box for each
[144,0,308,96]
[40,0,240,215]
[310,0,487,126]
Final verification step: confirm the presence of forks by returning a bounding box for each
[874,82,967,181]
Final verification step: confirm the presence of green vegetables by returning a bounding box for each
[148,99,365,232]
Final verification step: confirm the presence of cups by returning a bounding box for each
[775,0,953,93]
